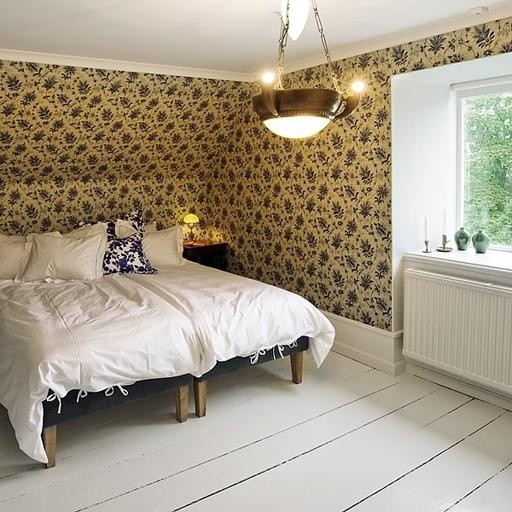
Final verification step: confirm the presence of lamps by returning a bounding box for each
[182,212,200,243]
[253,0,364,140]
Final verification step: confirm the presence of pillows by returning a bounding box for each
[79,209,158,275]
[63,222,106,237]
[12,230,107,283]
[141,223,186,268]
[141,220,157,232]
[0,231,59,281]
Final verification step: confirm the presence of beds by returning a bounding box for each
[1,209,332,468]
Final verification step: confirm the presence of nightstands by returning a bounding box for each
[182,237,226,269]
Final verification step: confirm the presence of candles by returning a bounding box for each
[442,208,446,234]
[424,215,429,240]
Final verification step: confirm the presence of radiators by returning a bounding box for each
[400,268,510,394]
